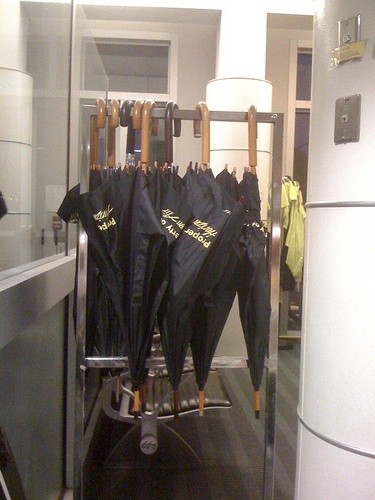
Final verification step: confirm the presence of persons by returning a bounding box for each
[1,190,30,500]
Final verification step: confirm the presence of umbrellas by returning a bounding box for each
[54,96,272,421]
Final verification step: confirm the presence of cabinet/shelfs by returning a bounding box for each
[70,103,286,500]
